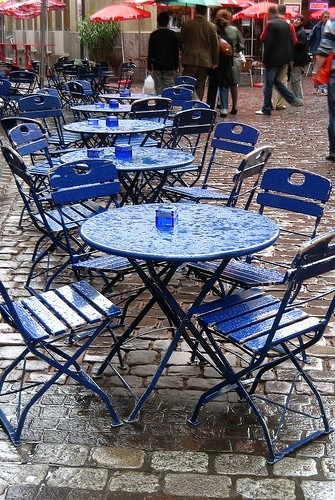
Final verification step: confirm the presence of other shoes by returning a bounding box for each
[230,110,237,115]
[255,109,265,115]
[326,153,335,162]
[219,108,228,117]
[312,87,328,96]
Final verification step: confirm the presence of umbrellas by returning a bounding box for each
[1,0,335,86]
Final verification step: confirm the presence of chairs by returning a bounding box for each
[0,55,335,467]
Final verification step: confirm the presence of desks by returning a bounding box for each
[58,146,195,294]
[97,93,160,119]
[79,203,280,424]
[70,104,167,149]
[62,120,166,206]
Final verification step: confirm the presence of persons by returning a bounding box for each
[147,5,335,162]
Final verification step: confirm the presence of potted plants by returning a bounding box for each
[76,7,121,77]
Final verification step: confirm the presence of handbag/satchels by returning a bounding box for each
[239,52,247,68]
[218,35,233,57]
[311,52,334,85]
[142,75,156,94]
